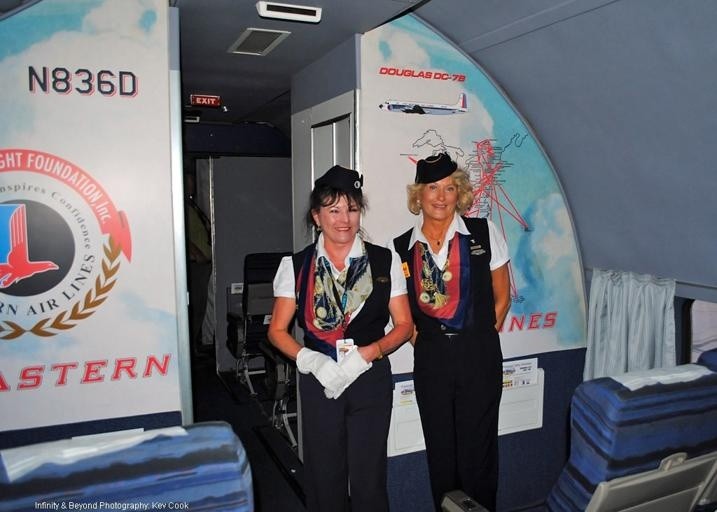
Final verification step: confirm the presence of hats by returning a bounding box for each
[314,164,365,192]
[415,151,458,186]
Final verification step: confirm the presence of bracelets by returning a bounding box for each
[373,341,383,360]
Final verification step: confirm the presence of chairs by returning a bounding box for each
[439,360,715,510]
[1,416,256,509]
[221,248,300,448]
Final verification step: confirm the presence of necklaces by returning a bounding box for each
[429,229,445,246]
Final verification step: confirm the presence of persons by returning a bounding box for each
[184,175,212,357]
[268,165,413,512]
[386,154,512,512]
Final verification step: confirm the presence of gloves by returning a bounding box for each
[296,344,374,402]
